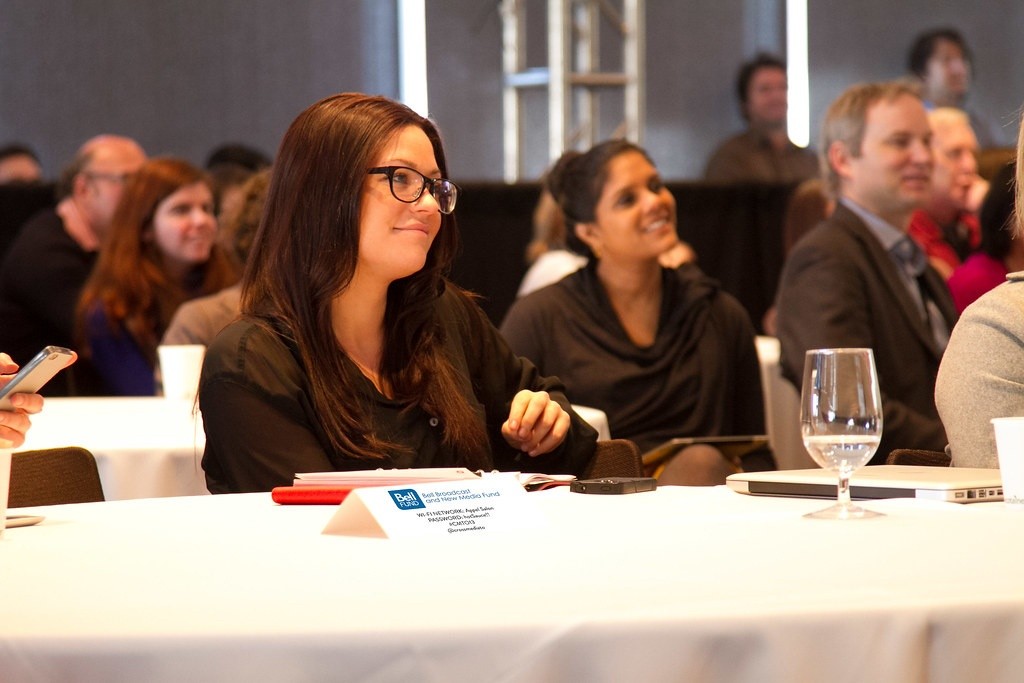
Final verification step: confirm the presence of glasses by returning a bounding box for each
[366,165,464,216]
[89,170,136,188]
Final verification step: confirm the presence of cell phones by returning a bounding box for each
[571,476,658,495]
[0,345,73,412]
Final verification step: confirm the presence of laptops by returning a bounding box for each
[726,464,1005,504]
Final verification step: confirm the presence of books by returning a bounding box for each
[272,467,482,504]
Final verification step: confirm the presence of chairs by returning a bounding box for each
[583,440,643,481]
[7,448,104,508]
[65,361,87,396]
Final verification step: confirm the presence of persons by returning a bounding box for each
[0,353,43,448]
[498,135,779,486]
[905,103,990,280]
[157,169,273,346]
[0,133,149,395]
[204,143,273,192]
[516,184,697,296]
[934,102,1024,469]
[905,25,1004,149]
[774,75,962,453]
[701,54,820,181]
[71,156,232,396]
[0,140,40,186]
[192,93,599,495]
[948,160,1017,312]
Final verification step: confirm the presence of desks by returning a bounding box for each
[0,394,614,510]
[0,481,1023,683]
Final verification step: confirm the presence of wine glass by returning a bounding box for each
[800,348,888,521]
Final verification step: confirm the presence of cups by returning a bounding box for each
[156,344,205,425]
[990,416,1024,517]
